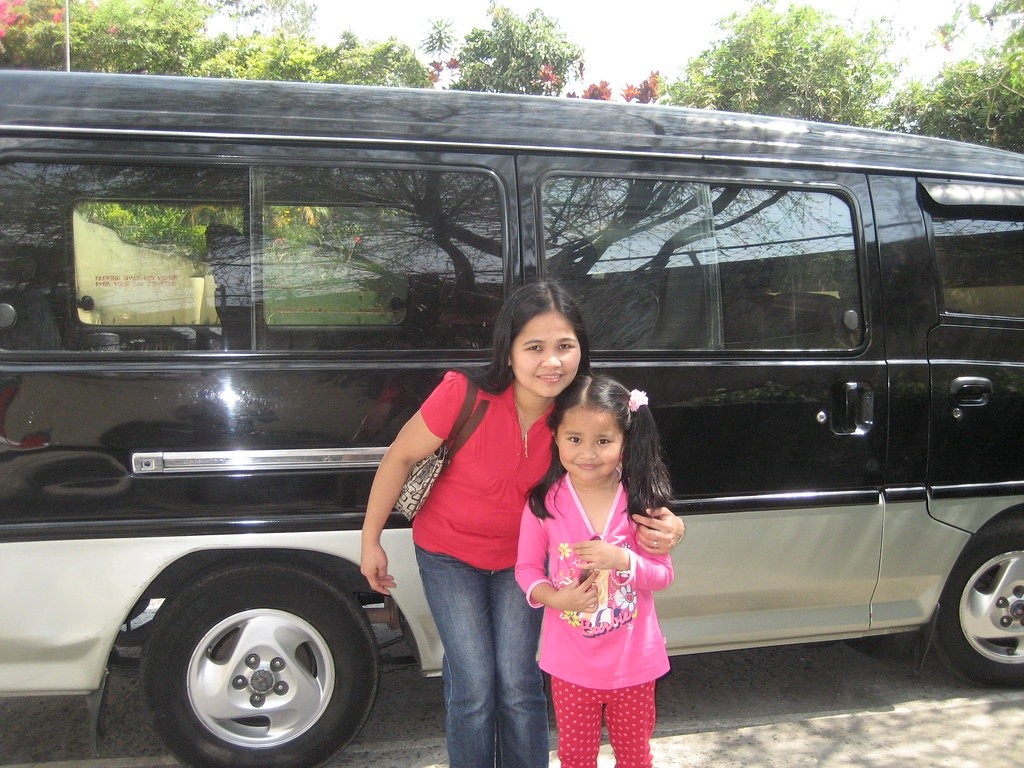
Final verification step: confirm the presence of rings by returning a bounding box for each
[653,540,657,548]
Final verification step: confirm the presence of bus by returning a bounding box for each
[1,69,1024,768]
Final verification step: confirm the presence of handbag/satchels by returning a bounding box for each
[394,377,491,520]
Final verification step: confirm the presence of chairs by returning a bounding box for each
[205,223,284,348]
[599,239,706,348]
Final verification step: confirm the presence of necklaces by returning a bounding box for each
[514,393,555,458]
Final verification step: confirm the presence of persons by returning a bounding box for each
[361,281,684,768]
[337,318,478,493]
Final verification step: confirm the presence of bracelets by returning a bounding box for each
[676,519,686,544]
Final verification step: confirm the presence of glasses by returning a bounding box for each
[578,533,602,585]
